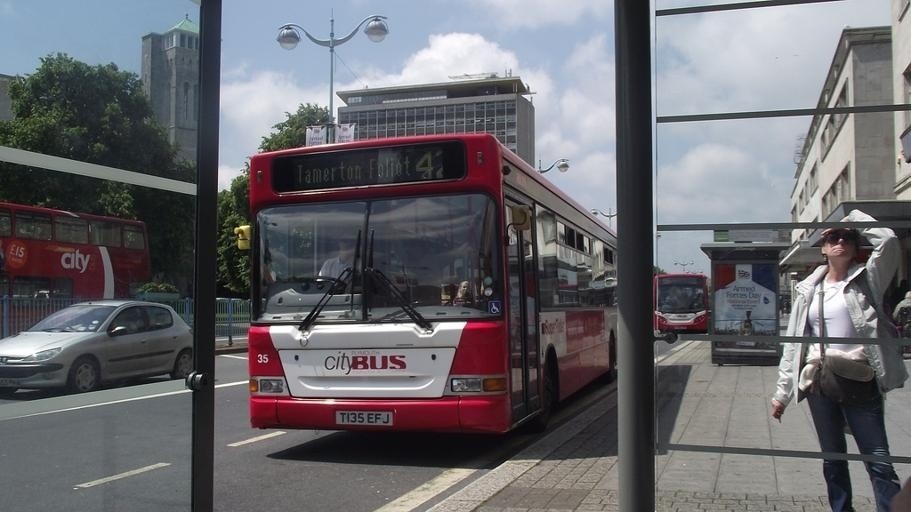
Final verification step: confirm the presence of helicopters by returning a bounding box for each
[715,310,776,336]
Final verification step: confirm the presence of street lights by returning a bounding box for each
[276,6,390,144]
[590,207,617,229]
[534,158,571,174]
[673,258,694,271]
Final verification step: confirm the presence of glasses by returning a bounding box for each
[822,230,857,245]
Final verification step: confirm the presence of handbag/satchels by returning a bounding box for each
[811,353,876,409]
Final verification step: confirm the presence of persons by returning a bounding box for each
[771,209,901,512]
[452,281,475,307]
[893,291,911,318]
[314,230,376,291]
[667,291,678,300]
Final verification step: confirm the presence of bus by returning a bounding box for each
[232,134,617,435]
[652,273,712,335]
[0,202,153,340]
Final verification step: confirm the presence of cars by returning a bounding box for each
[0,299,194,400]
